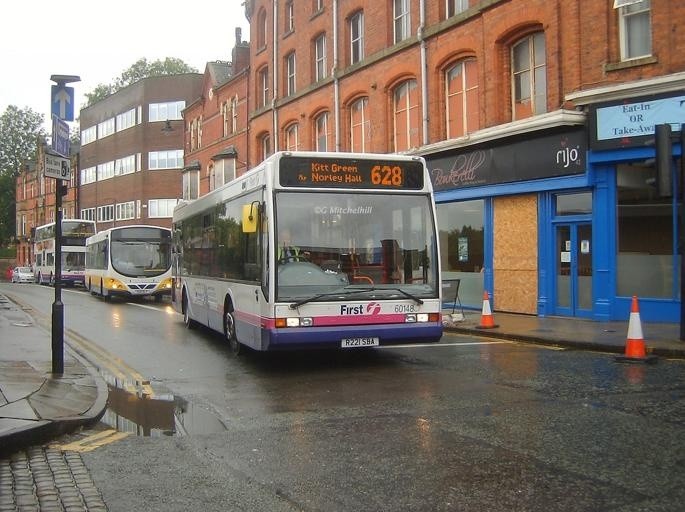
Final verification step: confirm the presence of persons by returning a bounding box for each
[277,228,310,263]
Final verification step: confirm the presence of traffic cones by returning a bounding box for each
[616,296,659,362]
[476,290,499,329]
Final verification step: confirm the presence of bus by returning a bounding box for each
[170,150,444,357]
[83,225,173,303]
[33,218,97,284]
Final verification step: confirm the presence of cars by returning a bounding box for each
[11,266,35,283]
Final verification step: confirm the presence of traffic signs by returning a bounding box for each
[44,154,71,181]
[52,114,70,158]
